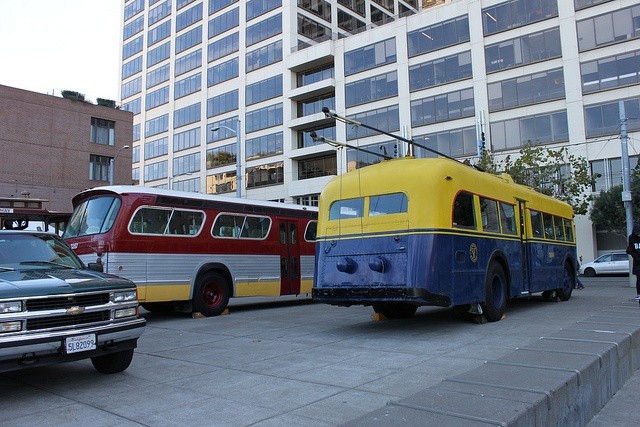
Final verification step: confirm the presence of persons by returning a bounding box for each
[626,224,640,299]
[577,259,584,290]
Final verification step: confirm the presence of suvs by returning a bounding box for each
[579,250,630,276]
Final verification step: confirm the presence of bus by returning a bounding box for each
[60,185,387,317]
[310,106,577,321]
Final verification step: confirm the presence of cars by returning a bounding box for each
[0,230,147,373]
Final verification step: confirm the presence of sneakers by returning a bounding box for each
[635,296,640,299]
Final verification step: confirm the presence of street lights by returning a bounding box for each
[211,118,246,198]
[110,145,129,185]
